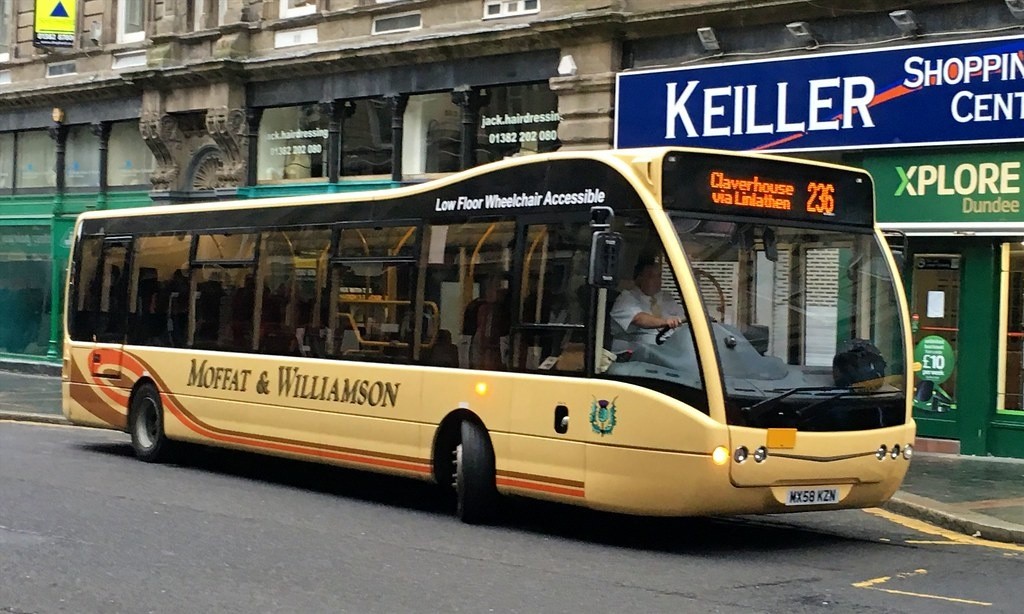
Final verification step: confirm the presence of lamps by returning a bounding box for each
[696,24,721,54]
[888,10,925,38]
[786,21,817,46]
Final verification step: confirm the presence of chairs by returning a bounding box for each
[427,330,459,369]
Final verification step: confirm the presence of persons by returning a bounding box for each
[229,250,576,368]
[608,259,691,363]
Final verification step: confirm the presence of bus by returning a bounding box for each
[57,144,922,523]
